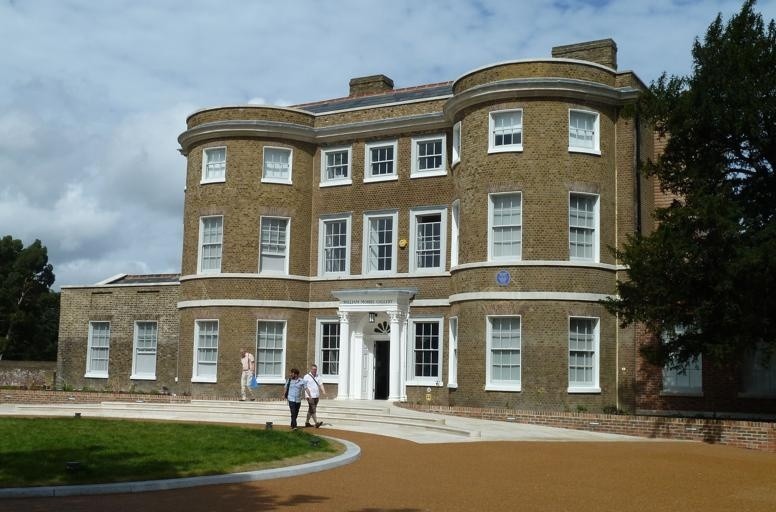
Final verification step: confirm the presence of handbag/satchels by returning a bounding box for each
[249,376,257,388]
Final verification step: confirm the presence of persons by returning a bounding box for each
[240,349,255,401]
[302,364,325,428]
[282,369,312,428]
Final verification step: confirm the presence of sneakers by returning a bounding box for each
[239,398,255,401]
[291,421,323,431]
